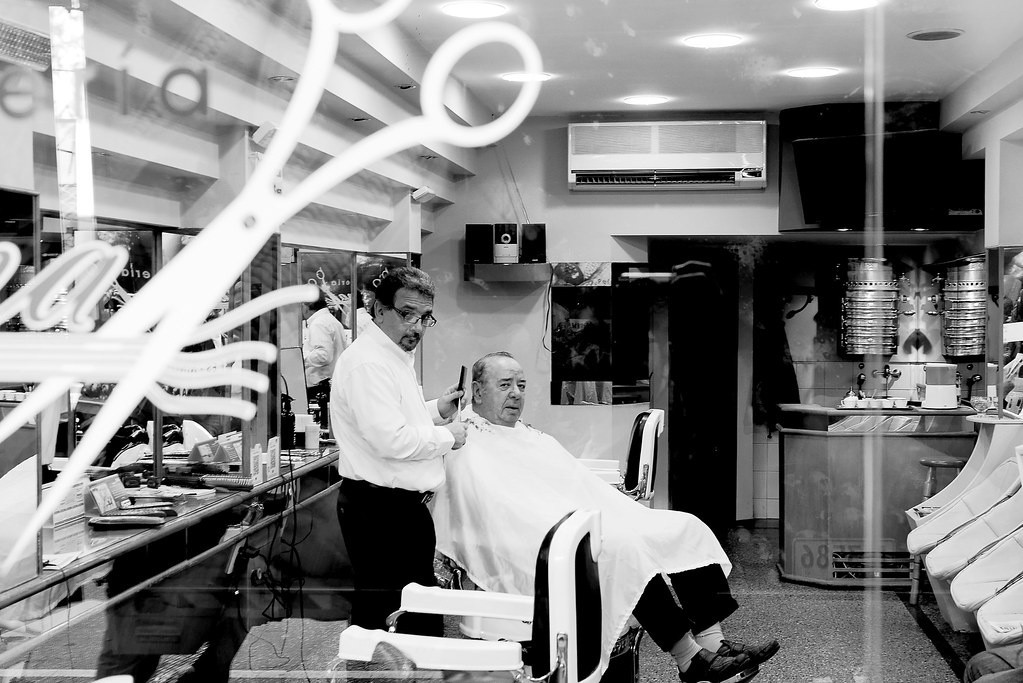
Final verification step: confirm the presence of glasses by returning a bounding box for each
[390,304,437,327]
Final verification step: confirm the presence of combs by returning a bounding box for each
[453,365,467,410]
[162,463,255,492]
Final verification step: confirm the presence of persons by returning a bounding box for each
[179,311,243,438]
[329,266,468,683]
[435,350,780,683]
[302,284,347,428]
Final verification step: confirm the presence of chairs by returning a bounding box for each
[576,408,665,508]
[339,506,602,683]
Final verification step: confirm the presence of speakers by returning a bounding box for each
[464,223,494,264]
[521,224,547,264]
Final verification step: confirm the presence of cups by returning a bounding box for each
[841,397,907,408]
[305,424,320,450]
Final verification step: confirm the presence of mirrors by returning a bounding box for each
[0,188,413,594]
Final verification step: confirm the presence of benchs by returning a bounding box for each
[906,456,1022,650]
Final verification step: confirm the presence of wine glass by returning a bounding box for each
[970,396,993,421]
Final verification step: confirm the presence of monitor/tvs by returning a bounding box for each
[791,129,965,225]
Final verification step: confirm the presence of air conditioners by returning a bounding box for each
[568,120,766,191]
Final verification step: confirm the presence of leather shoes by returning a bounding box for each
[716,640,780,665]
[676,648,751,683]
